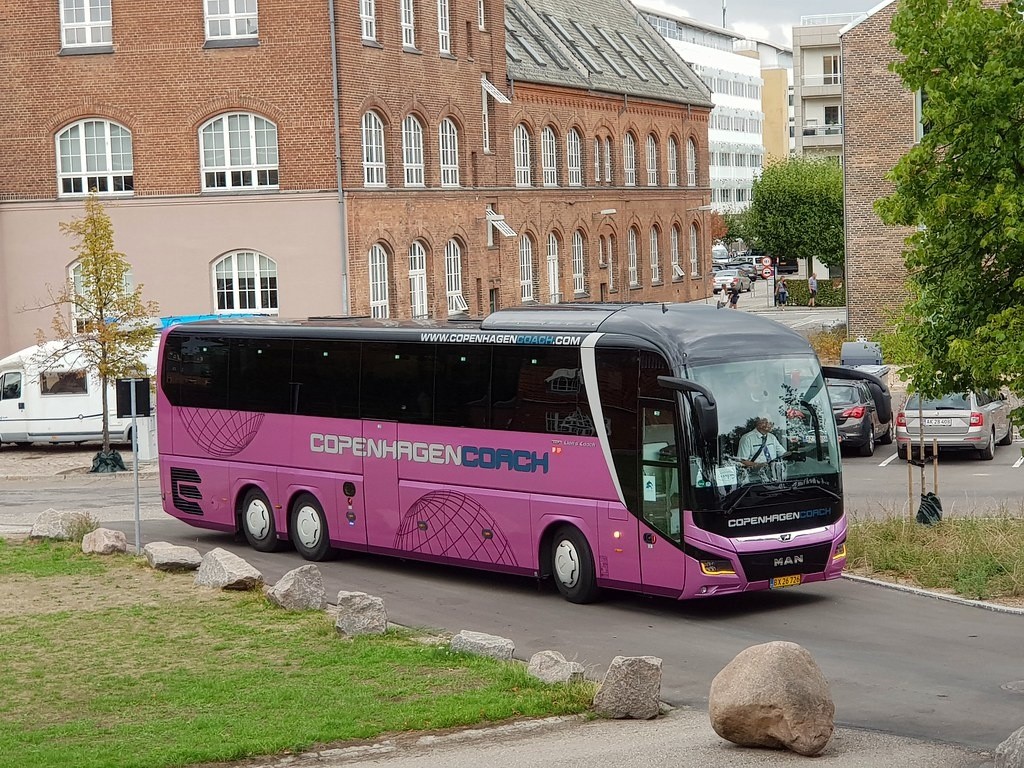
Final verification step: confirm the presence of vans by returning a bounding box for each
[0,331,163,449]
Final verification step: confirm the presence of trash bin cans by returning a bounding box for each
[840,364,891,387]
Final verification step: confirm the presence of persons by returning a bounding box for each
[736,408,792,470]
[773,276,788,310]
[716,283,740,310]
[808,274,819,307]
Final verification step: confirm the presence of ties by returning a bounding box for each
[761,436,772,463]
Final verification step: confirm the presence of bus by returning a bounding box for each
[156,299,849,605]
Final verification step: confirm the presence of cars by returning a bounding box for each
[713,268,753,295]
[792,378,894,457]
[709,241,798,282]
[895,380,1014,463]
